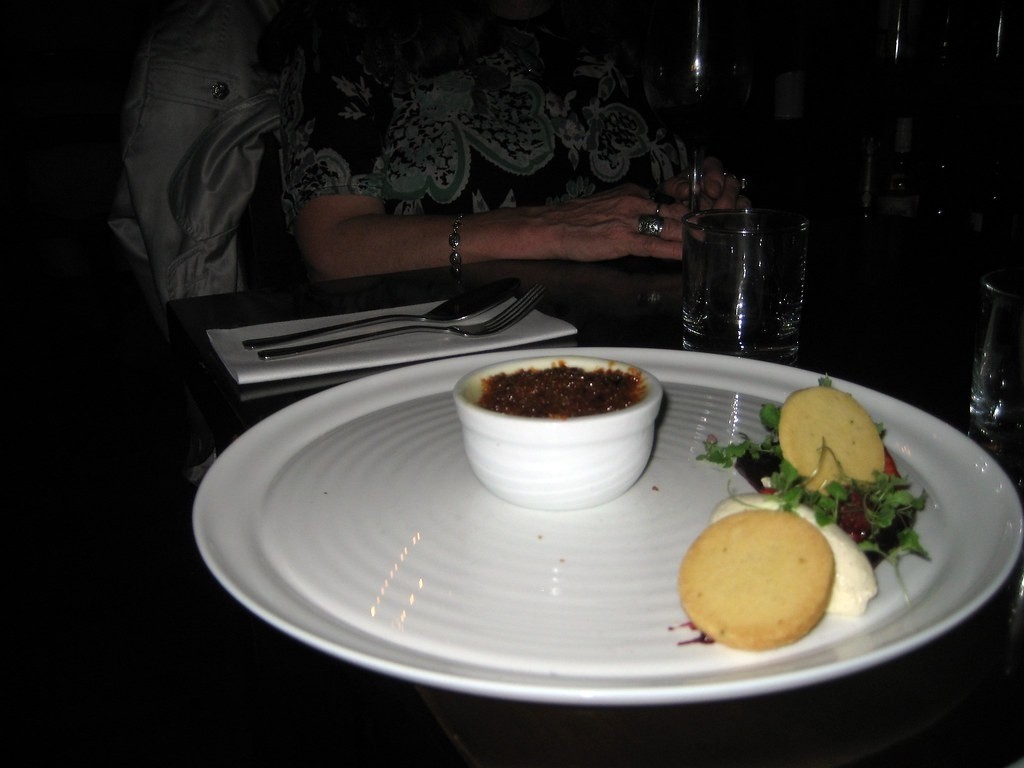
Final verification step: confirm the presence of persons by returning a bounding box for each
[105,1,752,302]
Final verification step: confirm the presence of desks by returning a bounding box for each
[166,223,1023,767]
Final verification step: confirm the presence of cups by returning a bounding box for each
[968,267,1024,445]
[453,355,663,512]
[680,209,810,368]
[875,0,1015,73]
[772,70,805,121]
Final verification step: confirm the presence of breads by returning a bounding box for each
[679,510,836,650]
[778,387,886,495]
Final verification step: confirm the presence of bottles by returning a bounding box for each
[855,118,1019,237]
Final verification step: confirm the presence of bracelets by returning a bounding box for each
[447,212,467,266]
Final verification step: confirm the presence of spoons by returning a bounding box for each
[242,276,522,350]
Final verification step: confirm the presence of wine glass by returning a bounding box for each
[640,0,750,213]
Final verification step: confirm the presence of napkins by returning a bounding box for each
[207,294,578,388]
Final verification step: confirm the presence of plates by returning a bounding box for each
[192,346,1024,707]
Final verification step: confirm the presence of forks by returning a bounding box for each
[256,283,547,362]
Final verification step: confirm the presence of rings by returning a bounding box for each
[649,191,675,217]
[638,214,665,238]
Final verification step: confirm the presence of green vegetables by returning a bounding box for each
[696,376,933,610]
